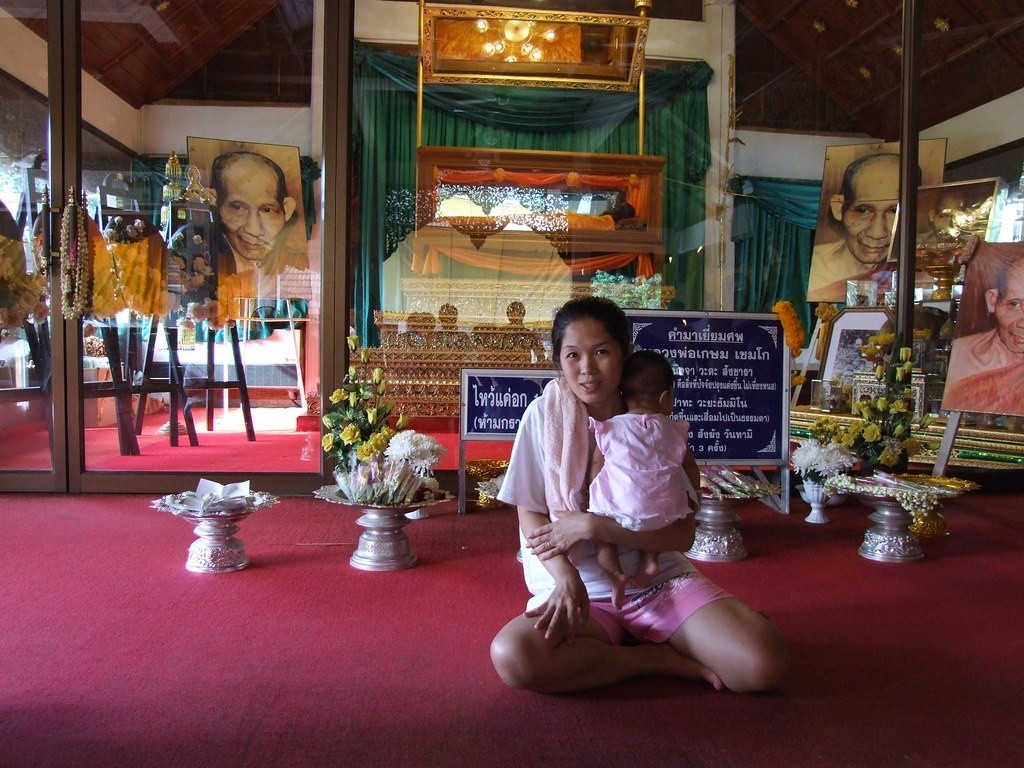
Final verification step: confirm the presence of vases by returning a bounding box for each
[313,485,456,571]
[804,483,830,524]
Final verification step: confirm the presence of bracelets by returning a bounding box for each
[695,489,703,494]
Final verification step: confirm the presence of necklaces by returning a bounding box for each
[61,201,93,320]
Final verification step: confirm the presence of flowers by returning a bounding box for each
[789,331,939,483]
[321,336,447,505]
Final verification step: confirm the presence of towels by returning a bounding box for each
[541,376,589,567]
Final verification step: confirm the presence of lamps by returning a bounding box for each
[162,151,186,226]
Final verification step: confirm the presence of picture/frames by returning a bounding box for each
[815,306,896,406]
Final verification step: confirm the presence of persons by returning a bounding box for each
[915,191,967,243]
[805,153,923,303]
[489,297,790,695]
[210,151,307,281]
[941,259,1024,416]
[847,339,867,371]
[397,303,545,364]
[587,350,702,609]
